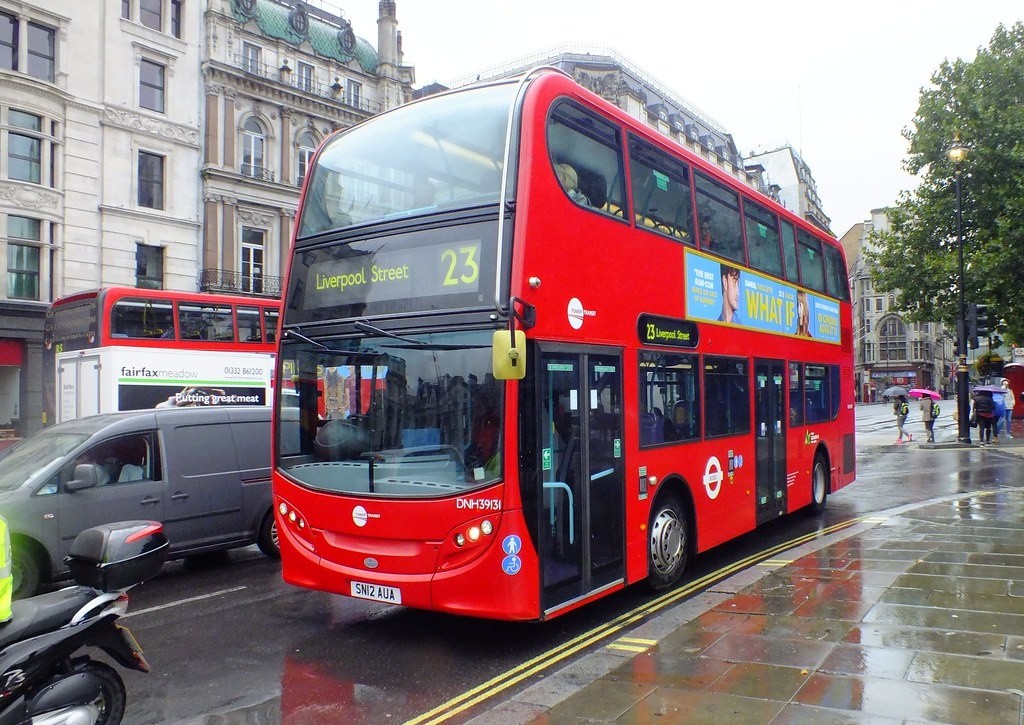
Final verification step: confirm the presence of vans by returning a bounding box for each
[0,407,307,601]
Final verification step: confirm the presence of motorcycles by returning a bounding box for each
[0,519,166,725]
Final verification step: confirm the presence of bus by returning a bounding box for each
[38,285,283,432]
[267,66,861,627]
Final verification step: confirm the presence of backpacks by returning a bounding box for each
[929,397,941,418]
[897,398,909,416]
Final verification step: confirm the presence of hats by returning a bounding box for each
[1000,377,1011,386]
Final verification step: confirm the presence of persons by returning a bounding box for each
[997,378,1015,439]
[919,393,936,442]
[973,391,998,445]
[664,400,711,444]
[894,395,912,443]
[691,221,722,254]
[59,440,110,491]
[794,289,813,339]
[717,263,741,324]
[649,407,676,443]
[553,163,587,205]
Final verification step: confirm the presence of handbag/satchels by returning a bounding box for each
[969,409,978,428]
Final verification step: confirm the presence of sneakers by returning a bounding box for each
[980,442,985,447]
[985,441,993,447]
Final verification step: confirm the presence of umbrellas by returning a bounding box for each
[882,386,908,396]
[973,385,1008,393]
[909,389,941,400]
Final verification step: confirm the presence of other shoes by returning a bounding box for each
[907,433,912,442]
[992,436,999,443]
[895,437,903,443]
[925,437,936,445]
[1006,434,1014,439]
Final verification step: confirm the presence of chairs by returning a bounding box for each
[41,141,852,547]
